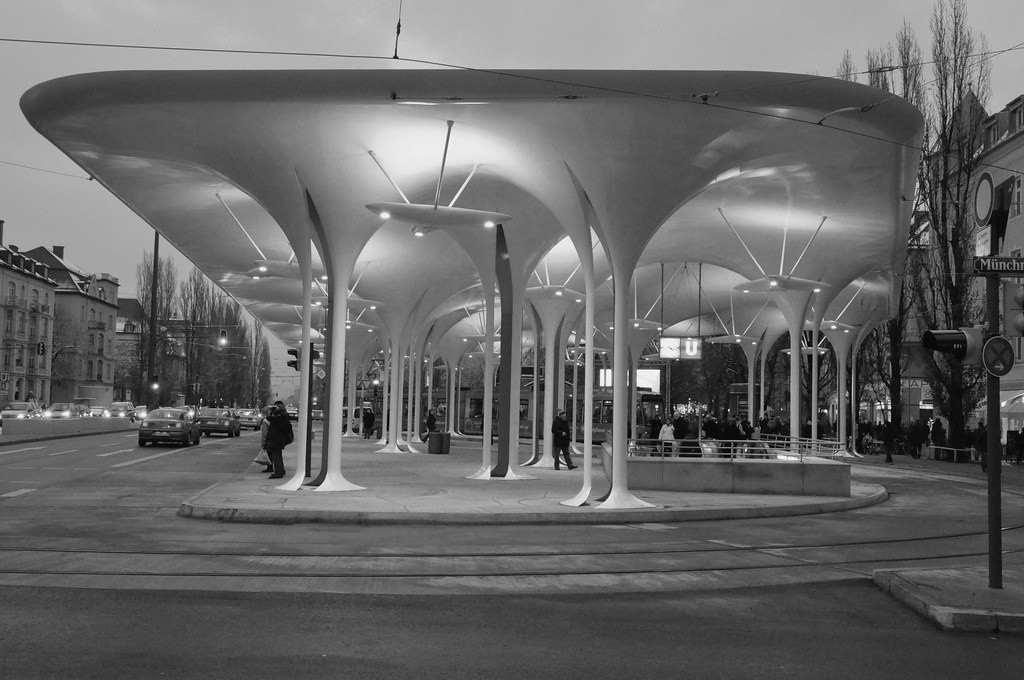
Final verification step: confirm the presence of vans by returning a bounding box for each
[108,402,135,423]
[343,407,374,434]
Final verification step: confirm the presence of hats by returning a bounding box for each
[268,405,279,416]
[558,408,566,416]
[274,400,285,407]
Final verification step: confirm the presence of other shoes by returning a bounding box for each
[421,439,425,443]
[270,471,285,475]
[269,473,283,479]
[364,436,367,439]
[555,468,561,470]
[263,468,273,472]
[368,437,370,439]
[569,466,578,470]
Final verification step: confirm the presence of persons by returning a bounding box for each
[261,400,290,479]
[649,413,755,457]
[551,410,578,471]
[422,409,436,443]
[759,413,831,440]
[833,417,1024,473]
[363,408,375,439]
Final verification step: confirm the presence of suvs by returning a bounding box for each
[285,407,299,422]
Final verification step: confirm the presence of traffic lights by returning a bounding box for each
[39,343,43,355]
[220,329,228,347]
[920,326,985,366]
[287,347,300,371]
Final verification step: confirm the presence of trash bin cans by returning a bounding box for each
[377,425,383,438]
[428,431,451,454]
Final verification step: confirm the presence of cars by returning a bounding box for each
[0,402,44,420]
[237,408,261,430]
[134,406,147,421]
[311,409,324,421]
[162,405,210,420]
[43,402,110,418]
[138,409,201,447]
[198,408,241,438]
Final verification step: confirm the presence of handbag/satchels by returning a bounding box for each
[254,448,272,465]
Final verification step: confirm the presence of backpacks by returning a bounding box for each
[278,412,294,444]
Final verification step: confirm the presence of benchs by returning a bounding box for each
[873,441,904,454]
[928,445,976,462]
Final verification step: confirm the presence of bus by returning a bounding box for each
[375,370,665,447]
